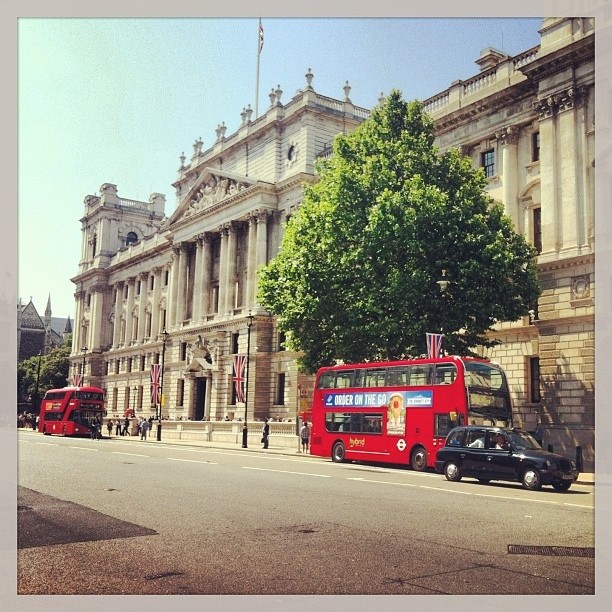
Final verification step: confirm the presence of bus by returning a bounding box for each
[309,356,514,473]
[37,386,107,437]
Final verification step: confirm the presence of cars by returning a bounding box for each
[434,424,580,492]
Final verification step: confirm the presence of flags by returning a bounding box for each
[72,374,83,386]
[425,332,444,358]
[230,353,247,404]
[149,364,162,404]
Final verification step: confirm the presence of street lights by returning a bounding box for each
[32,349,43,430]
[80,346,89,387]
[240,308,255,448]
[155,329,170,441]
[435,268,451,357]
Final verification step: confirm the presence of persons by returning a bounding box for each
[299,422,311,454]
[494,435,506,449]
[91,417,152,441]
[262,419,269,449]
